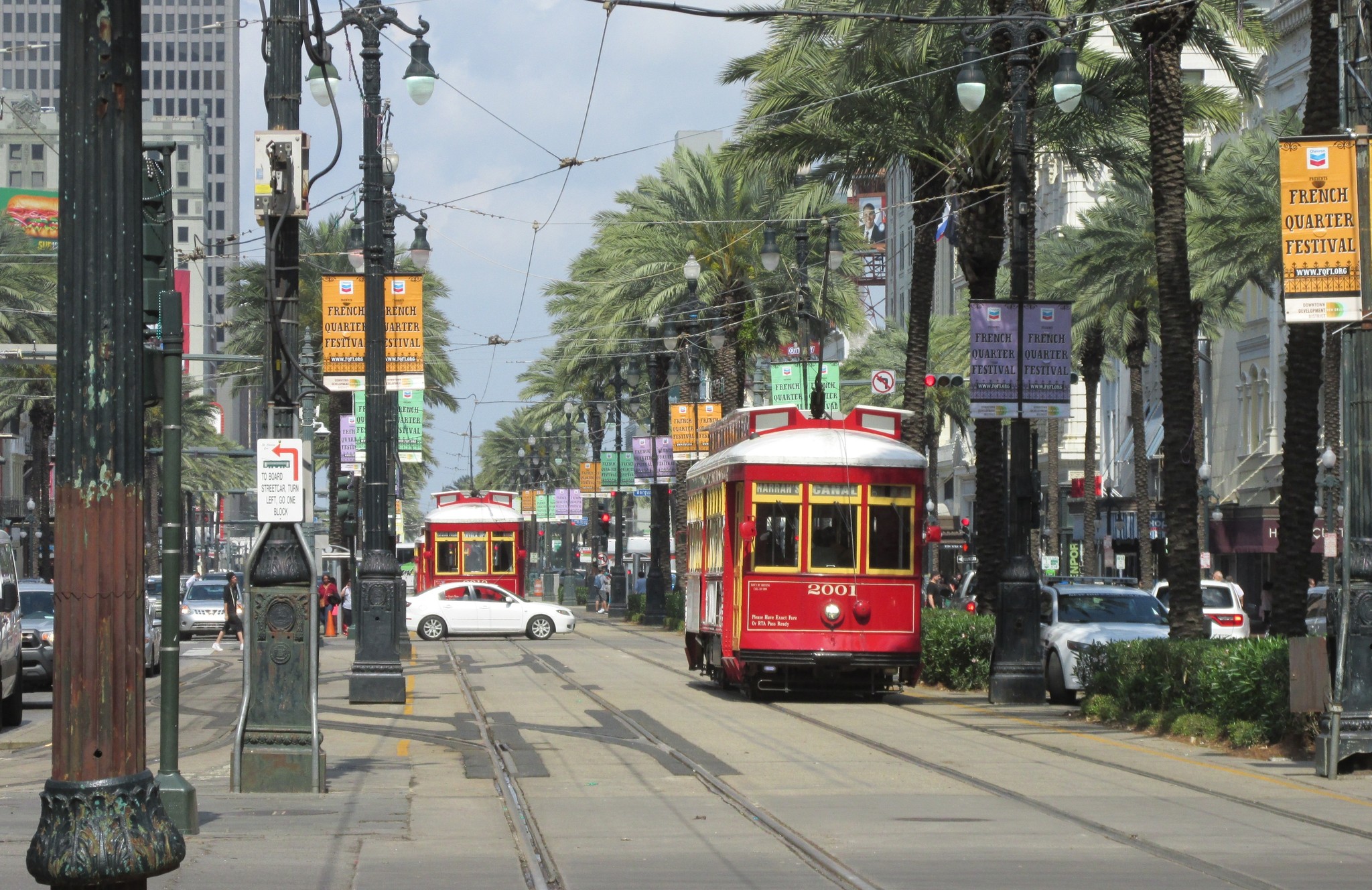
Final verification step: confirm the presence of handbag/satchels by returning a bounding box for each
[319,599,325,608]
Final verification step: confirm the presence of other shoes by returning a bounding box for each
[345,633,347,637]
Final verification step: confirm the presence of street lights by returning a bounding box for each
[511,351,642,620]
[304,0,440,703]
[1197,464,1224,579]
[954,2,1083,703]
[1314,439,1344,699]
[922,498,943,580]
[638,246,728,626]
[349,131,432,660]
[759,211,844,409]
[1094,471,1125,584]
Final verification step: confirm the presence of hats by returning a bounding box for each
[929,570,939,580]
[226,572,235,580]
[604,572,610,575]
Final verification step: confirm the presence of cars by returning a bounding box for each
[145,609,162,677]
[405,582,575,641]
[1264,585,1329,637]
[1152,580,1251,644]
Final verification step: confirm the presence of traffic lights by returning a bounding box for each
[1071,372,1079,385]
[961,516,972,554]
[926,372,965,388]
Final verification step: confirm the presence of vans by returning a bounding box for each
[0,529,22,729]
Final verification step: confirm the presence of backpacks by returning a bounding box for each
[593,574,605,589]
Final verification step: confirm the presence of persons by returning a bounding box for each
[50,577,54,584]
[594,566,612,615]
[318,574,352,636]
[185,572,200,588]
[475,589,482,599]
[1213,570,1228,584]
[237,658,243,662]
[212,572,244,651]
[1226,575,1244,608]
[1261,582,1272,620]
[634,571,646,594]
[921,570,962,610]
[859,202,885,245]
[1309,578,1316,589]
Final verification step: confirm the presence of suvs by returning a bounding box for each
[204,571,244,603]
[950,569,978,617]
[1039,572,1172,704]
[14,578,54,692]
[145,574,162,619]
[180,580,244,641]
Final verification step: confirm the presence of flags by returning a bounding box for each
[807,362,840,411]
[522,488,583,522]
[935,200,951,241]
[670,404,696,460]
[655,436,677,484]
[580,462,595,498]
[384,276,425,391]
[354,391,367,462]
[396,389,423,463]
[632,437,654,485]
[596,462,611,498]
[770,363,805,410]
[619,452,637,491]
[340,414,363,471]
[600,451,618,492]
[321,276,365,392]
[968,302,1020,419]
[1277,140,1364,323]
[698,403,722,460]
[1019,304,1073,419]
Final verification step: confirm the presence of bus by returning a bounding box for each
[396,542,415,590]
[413,489,527,601]
[684,402,929,704]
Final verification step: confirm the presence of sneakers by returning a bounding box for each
[604,610,608,614]
[595,610,598,613]
[598,608,604,613]
[212,643,223,652]
[240,643,243,650]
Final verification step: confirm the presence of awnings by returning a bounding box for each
[1207,517,1345,553]
[1068,510,1168,539]
